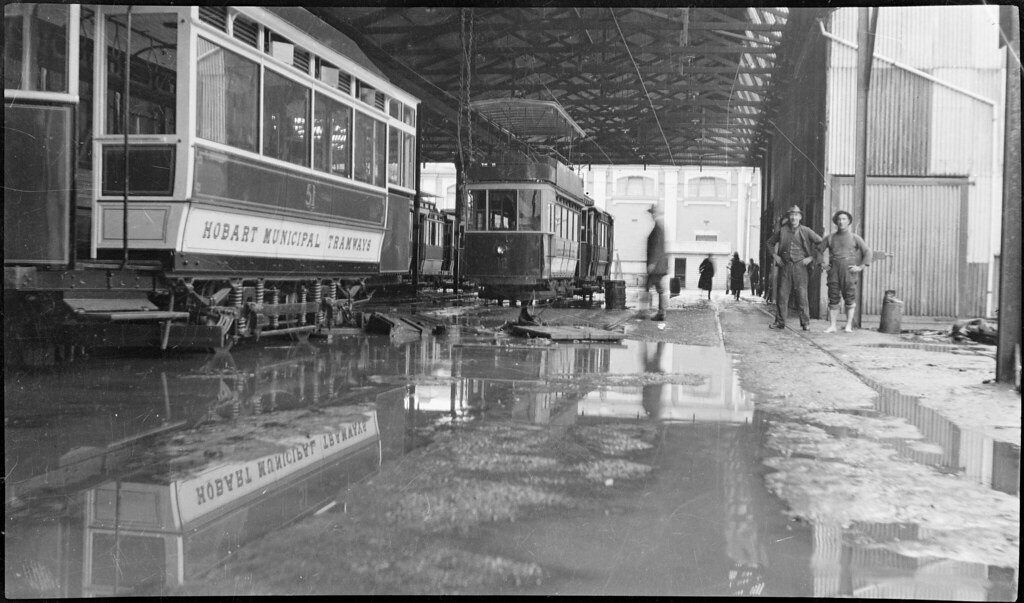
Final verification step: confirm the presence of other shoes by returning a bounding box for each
[768,322,785,330]
[803,324,810,331]
[651,313,666,321]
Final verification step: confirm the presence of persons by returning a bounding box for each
[815,209,873,333]
[630,201,670,321]
[766,205,824,330]
[747,259,760,296]
[727,251,746,300]
[698,253,714,301]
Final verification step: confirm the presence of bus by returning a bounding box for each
[401,196,464,294]
[3,3,421,353]
[460,98,615,306]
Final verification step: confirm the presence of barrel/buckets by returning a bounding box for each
[605,280,626,309]
[670,277,681,296]
[877,302,903,334]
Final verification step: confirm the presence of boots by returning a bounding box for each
[845,302,856,332]
[823,302,841,334]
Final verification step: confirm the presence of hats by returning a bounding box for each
[786,206,804,216]
[832,209,852,226]
[646,204,664,214]
